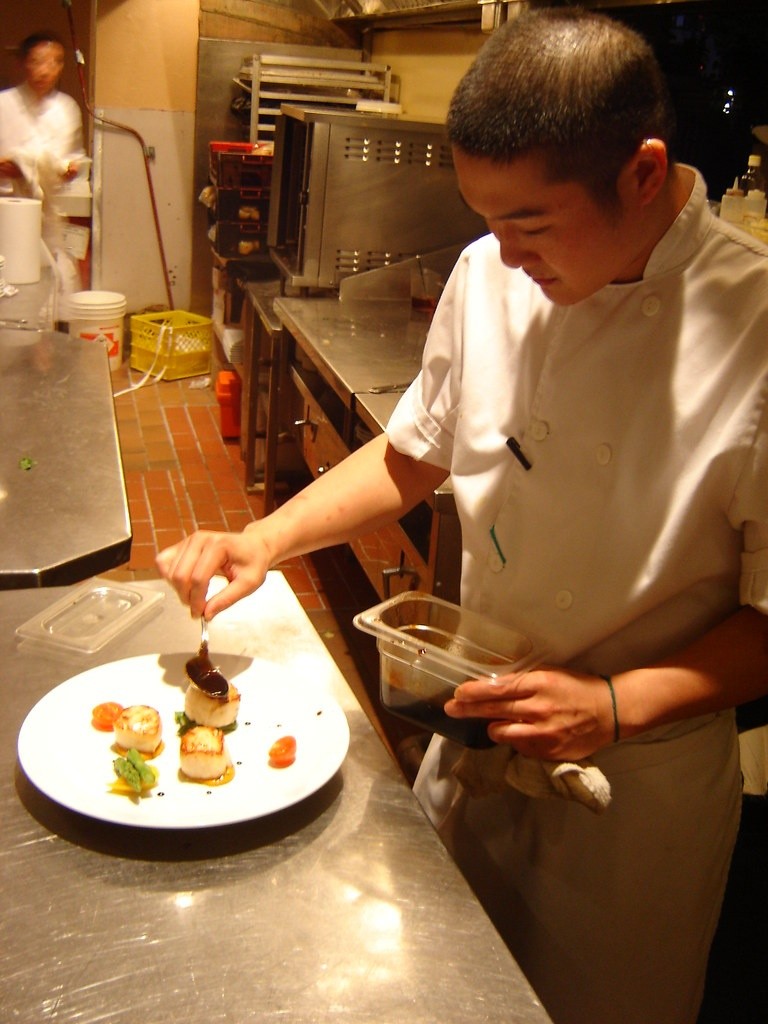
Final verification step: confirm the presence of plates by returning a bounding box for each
[17,651,350,829]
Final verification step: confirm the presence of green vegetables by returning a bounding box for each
[174,712,239,736]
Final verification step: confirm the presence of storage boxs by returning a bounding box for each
[209,147,274,257]
[218,370,242,439]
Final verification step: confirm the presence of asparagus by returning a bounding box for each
[111,748,157,794]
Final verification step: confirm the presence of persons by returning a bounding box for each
[156,11,768,1024]
[0,31,89,293]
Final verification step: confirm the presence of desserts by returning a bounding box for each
[113,704,162,752]
[178,726,228,780]
[184,679,241,729]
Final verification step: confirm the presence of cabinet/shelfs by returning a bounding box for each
[238,53,392,144]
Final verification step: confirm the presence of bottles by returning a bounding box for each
[719,176,744,223]
[744,190,767,219]
[740,154,765,197]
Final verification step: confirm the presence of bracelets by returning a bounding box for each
[605,676,618,748]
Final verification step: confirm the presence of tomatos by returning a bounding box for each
[91,702,124,728]
[268,736,297,766]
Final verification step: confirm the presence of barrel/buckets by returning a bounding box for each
[67,291,126,371]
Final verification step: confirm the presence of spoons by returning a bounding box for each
[185,612,229,697]
